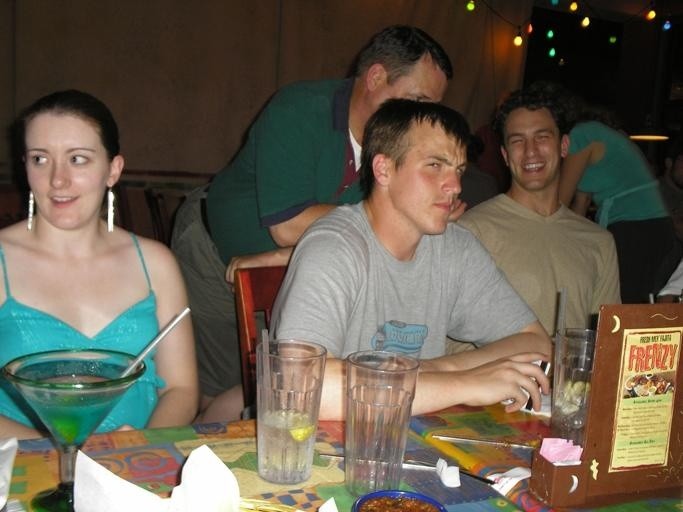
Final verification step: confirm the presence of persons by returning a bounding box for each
[173,26,621,424]
[653,141,682,294]
[559,90,674,302]
[0,90,200,442]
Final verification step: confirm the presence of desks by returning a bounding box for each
[0,418,524,511]
[409,396,585,512]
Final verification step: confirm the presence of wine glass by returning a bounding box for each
[2,347,146,511]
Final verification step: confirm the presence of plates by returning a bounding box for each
[353,491,449,511]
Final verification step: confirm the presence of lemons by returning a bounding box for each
[290,418,315,442]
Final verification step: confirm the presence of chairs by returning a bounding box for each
[234,264,291,420]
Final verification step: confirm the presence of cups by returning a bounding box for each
[344,351,419,500]
[552,327,598,448]
[254,339,327,482]
